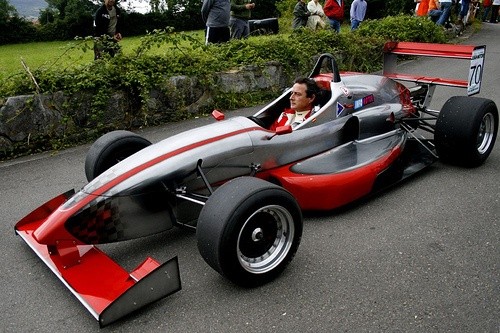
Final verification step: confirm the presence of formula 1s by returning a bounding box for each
[14,41,499,328]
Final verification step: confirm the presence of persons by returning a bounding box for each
[91,0,122,60]
[201,0,500,45]
[270,77,323,130]
